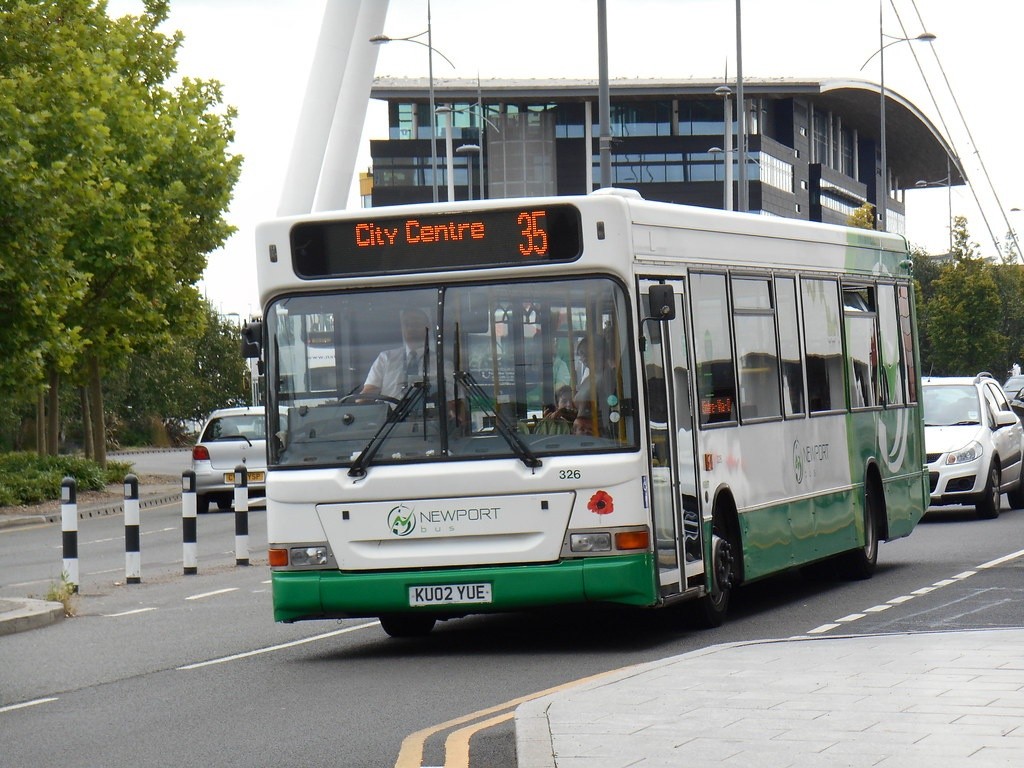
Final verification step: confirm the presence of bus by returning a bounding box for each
[251,186,927,636]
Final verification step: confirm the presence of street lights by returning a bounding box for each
[710,84,735,210]
[876,0,937,231]
[367,0,438,204]
[455,143,480,201]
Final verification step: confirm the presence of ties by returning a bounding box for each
[406,351,418,376]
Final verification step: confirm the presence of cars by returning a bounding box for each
[920,372,1024,515]
[188,404,270,514]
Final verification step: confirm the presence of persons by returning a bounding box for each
[541,326,618,438]
[354,307,466,426]
